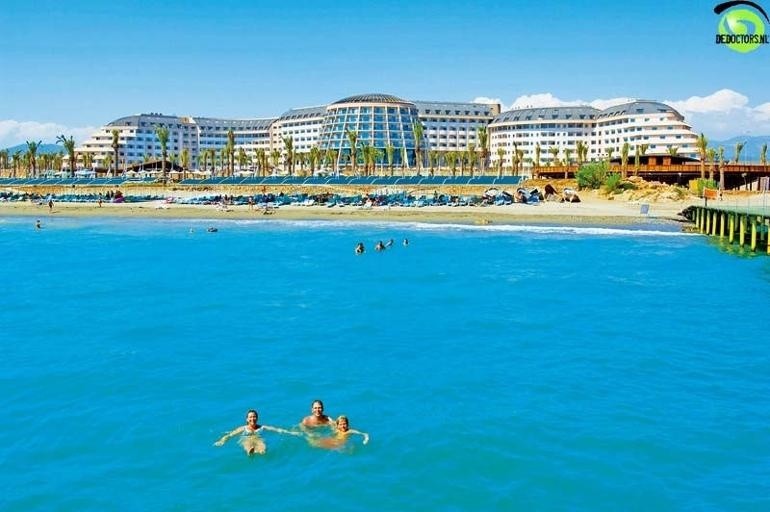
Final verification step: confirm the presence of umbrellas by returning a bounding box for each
[54,171,71,177]
[125,170,213,180]
[75,170,97,175]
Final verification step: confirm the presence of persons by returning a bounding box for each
[300,400,337,438]
[303,415,369,450]
[214,409,299,457]
[98,193,102,207]
[248,195,254,210]
[36,219,40,230]
[218,193,235,205]
[363,193,385,206]
[49,199,54,210]
[514,191,523,203]
[105,190,124,203]
[355,236,410,255]
